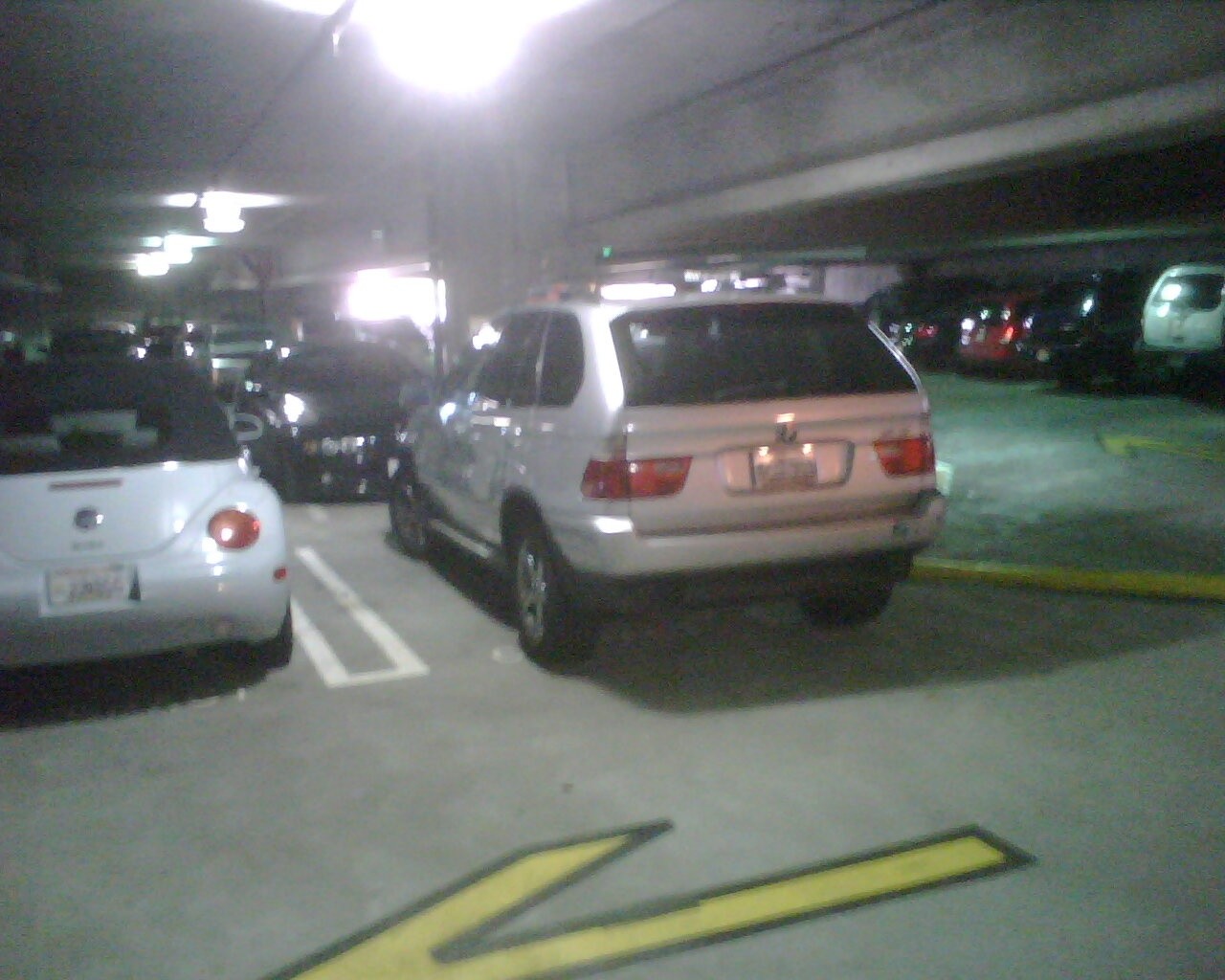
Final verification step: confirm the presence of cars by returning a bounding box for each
[0,317,442,505]
[867,274,1059,381]
[0,355,294,689]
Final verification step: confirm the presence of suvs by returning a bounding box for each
[1137,260,1225,399]
[1013,270,1157,393]
[379,289,950,668]
